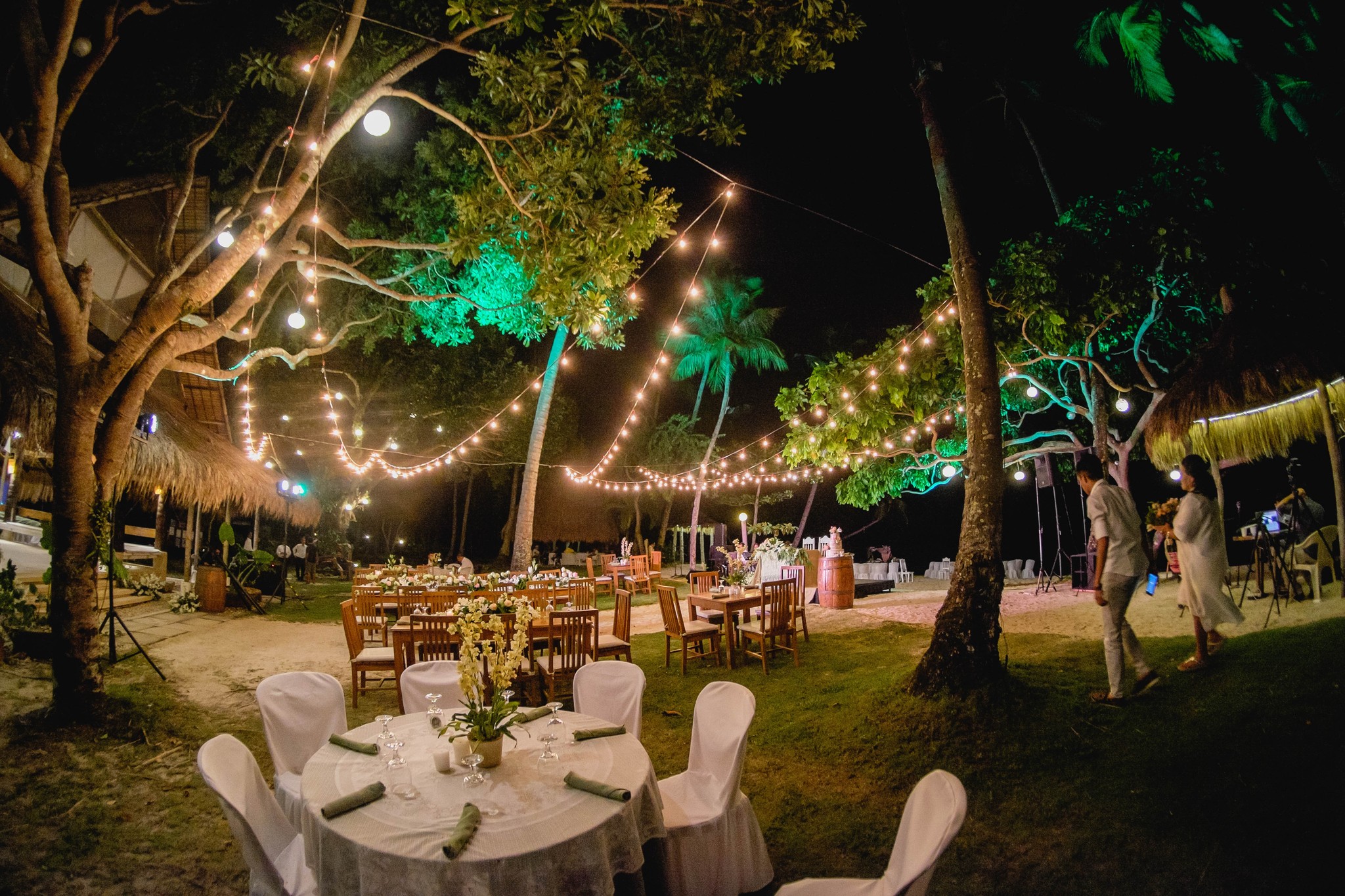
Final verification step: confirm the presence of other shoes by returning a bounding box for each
[1129,670,1159,697]
[1091,691,1122,708]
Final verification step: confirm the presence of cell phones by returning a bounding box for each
[1145,573,1158,597]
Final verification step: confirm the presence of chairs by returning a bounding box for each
[196,732,318,896]
[571,655,648,740]
[341,538,815,714]
[639,678,774,895]
[854,557,1036,584]
[255,672,353,821]
[773,768,971,896]
[1288,525,1342,601]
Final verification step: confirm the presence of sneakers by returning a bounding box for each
[1208,639,1224,656]
[1177,657,1209,671]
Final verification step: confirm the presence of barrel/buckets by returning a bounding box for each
[818,554,855,610]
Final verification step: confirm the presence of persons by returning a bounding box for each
[456,553,475,580]
[276,539,291,578]
[532,540,666,568]
[332,550,349,581]
[735,540,831,572]
[293,536,309,581]
[240,530,254,585]
[1236,487,1328,601]
[305,538,319,585]
[1156,453,1246,672]
[1077,454,1161,708]
[257,537,264,550]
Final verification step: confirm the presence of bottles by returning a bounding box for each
[374,714,398,763]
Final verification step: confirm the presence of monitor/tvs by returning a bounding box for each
[1255,510,1280,533]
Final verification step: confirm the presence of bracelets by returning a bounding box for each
[1165,531,1171,540]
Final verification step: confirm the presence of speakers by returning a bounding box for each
[686,570,710,584]
[1073,445,1097,464]
[1071,553,1097,592]
[1033,451,1060,489]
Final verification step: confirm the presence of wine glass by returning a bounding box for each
[500,688,518,725]
[754,577,761,589]
[383,740,412,795]
[413,604,421,615]
[421,607,430,616]
[535,732,563,782]
[546,701,567,743]
[425,692,445,735]
[734,581,741,598]
[718,580,725,594]
[460,752,491,811]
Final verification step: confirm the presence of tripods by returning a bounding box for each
[98,474,166,681]
[265,496,307,610]
[212,552,267,616]
[1033,476,1101,596]
[1238,484,1345,630]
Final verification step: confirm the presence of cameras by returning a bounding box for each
[1285,456,1303,478]
